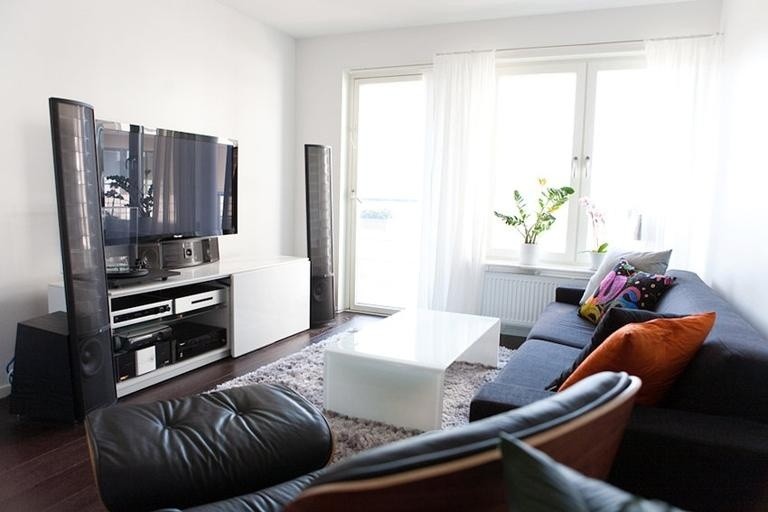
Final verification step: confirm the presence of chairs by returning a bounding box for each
[158,369,643,511]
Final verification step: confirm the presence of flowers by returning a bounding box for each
[578,195,612,254]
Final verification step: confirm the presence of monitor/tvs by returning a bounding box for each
[92,118,239,247]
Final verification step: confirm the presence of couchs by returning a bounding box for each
[468,268,768,512]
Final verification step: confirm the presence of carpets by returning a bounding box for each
[200,325,519,467]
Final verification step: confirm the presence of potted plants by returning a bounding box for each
[492,175,576,265]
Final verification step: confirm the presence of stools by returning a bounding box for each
[83,380,335,511]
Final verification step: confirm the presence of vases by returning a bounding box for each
[587,251,606,271]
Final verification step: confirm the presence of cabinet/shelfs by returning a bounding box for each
[47,251,312,401]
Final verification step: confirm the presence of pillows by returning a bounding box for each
[543,247,717,408]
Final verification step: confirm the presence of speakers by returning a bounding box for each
[310,274,336,324]
[130,243,163,272]
[10,310,117,426]
[202,237,220,263]
[163,238,204,269]
